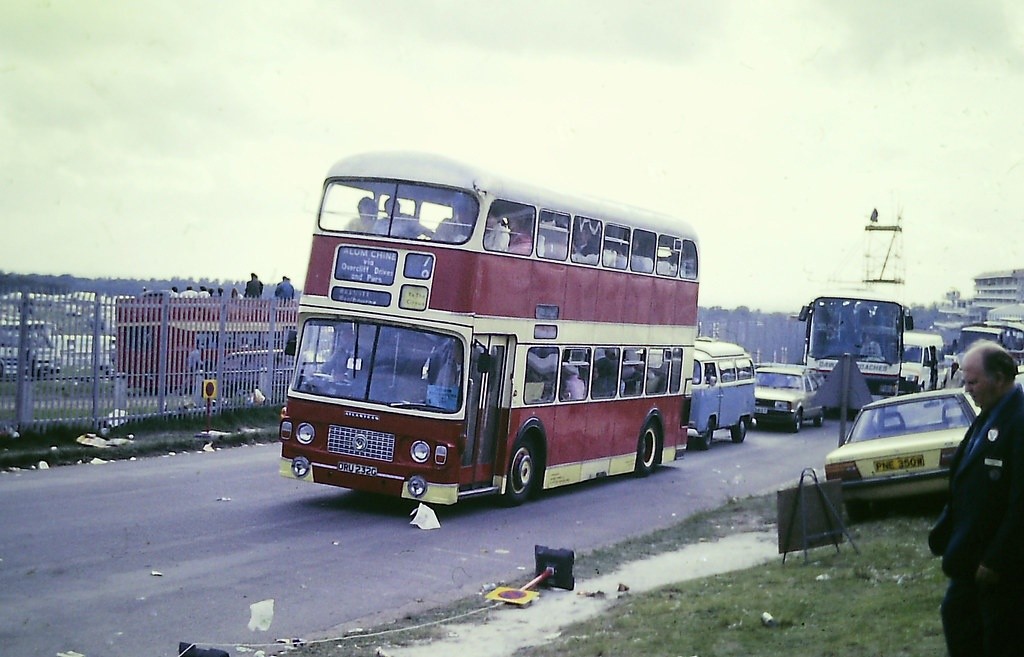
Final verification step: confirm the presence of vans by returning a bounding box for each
[901,318,1024,394]
[689,337,757,446]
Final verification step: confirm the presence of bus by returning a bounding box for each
[115,294,299,387]
[794,297,914,412]
[0,316,115,380]
[280,151,701,505]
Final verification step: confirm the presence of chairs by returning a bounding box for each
[879,410,905,436]
[499,228,686,277]
[942,400,968,424]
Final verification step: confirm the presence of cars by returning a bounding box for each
[747,364,830,430]
[822,390,983,523]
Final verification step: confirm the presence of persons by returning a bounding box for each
[344,198,696,277]
[860,335,881,362]
[928,339,1024,657]
[170,273,294,299]
[943,363,960,392]
[310,325,683,405]
